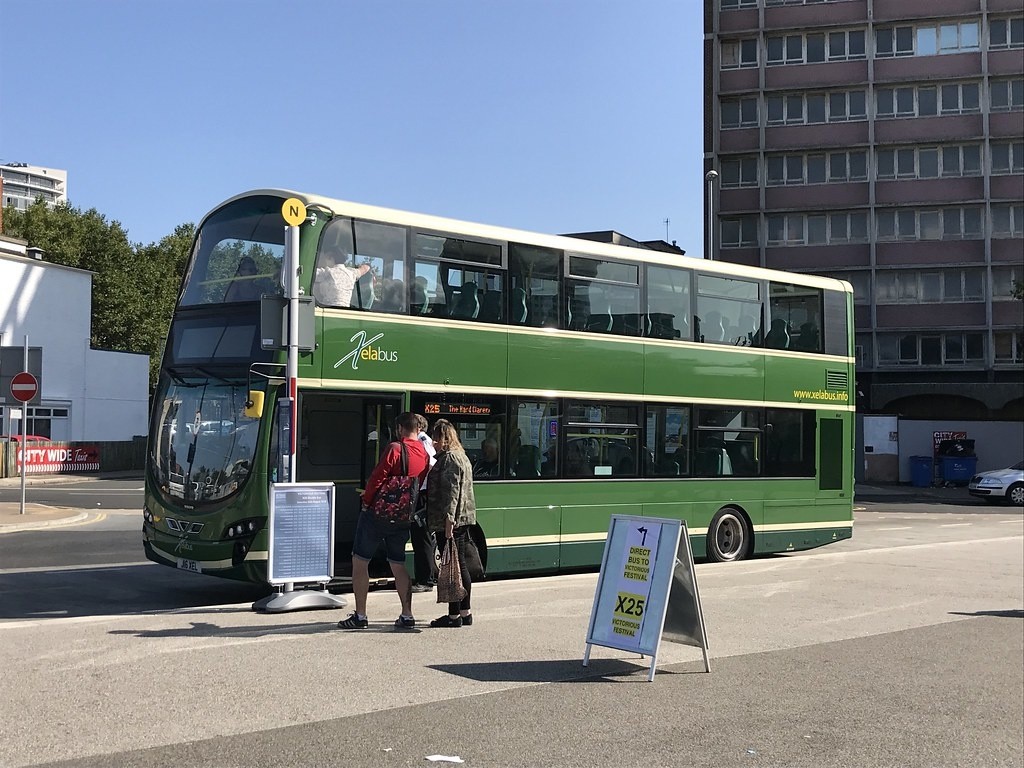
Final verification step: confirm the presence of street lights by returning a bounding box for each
[706,170,719,260]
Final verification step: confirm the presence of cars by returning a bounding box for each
[0,435,54,447]
[155,420,289,464]
[968,461,1024,507]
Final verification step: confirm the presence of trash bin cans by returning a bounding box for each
[937,439,978,488]
[911,456,934,488]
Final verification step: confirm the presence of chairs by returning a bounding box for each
[351,265,821,353]
[515,445,735,479]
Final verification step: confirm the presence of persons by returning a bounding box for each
[316,245,370,307]
[569,445,592,475]
[412,414,437,592]
[426,419,476,627]
[228,258,262,300]
[238,420,278,463]
[472,439,498,475]
[338,413,429,629]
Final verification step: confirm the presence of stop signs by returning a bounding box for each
[10,373,37,403]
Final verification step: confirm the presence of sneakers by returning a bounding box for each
[462,614,472,625]
[395,615,415,628]
[338,610,368,628]
[431,615,462,627]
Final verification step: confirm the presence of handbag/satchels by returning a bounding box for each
[463,541,484,582]
[437,536,467,603]
[372,476,418,527]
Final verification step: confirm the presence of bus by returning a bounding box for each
[143,187,857,594]
